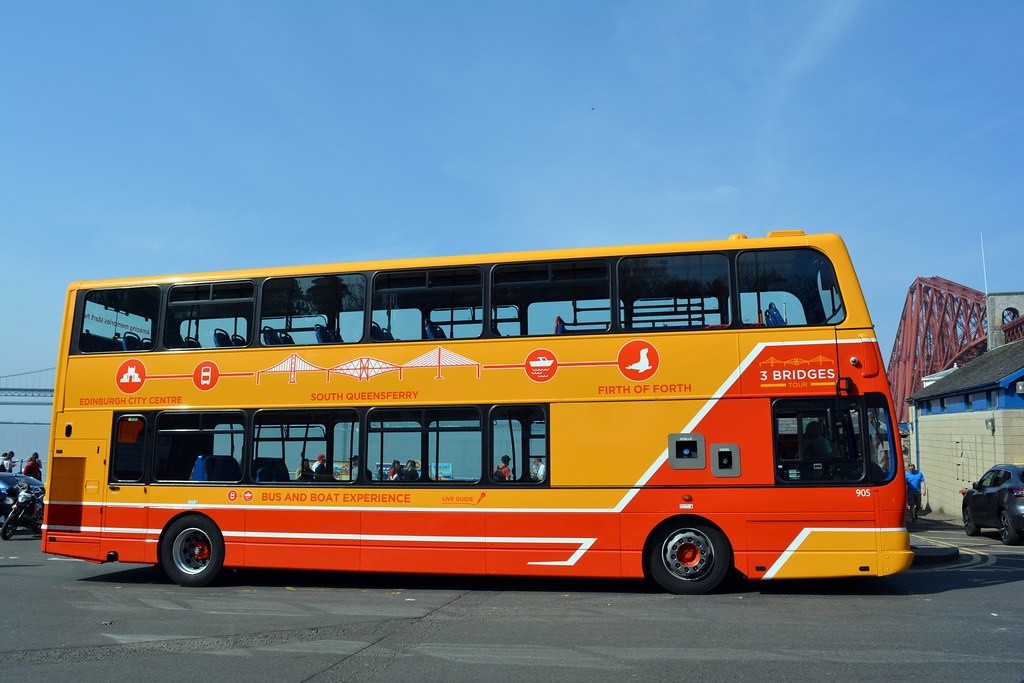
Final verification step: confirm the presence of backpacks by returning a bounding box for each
[493,465,508,481]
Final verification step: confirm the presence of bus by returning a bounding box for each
[42,230,913,595]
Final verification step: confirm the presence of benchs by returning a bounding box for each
[764,301,786,326]
[123,316,568,352]
[79,332,123,353]
[188,455,290,482]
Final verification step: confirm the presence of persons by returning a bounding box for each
[533,458,546,482]
[296,454,326,481]
[493,455,511,480]
[23,452,42,481]
[905,464,926,522]
[869,433,890,472]
[0,451,18,473]
[795,421,833,480]
[390,458,419,480]
[349,454,359,481]
[376,467,380,479]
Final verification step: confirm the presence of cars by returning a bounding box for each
[0,471,46,528]
[959,462,1024,546]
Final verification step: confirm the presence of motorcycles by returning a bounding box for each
[0,474,45,540]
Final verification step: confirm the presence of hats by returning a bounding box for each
[349,455,359,460]
[501,455,512,461]
[318,454,325,461]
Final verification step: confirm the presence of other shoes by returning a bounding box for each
[912,518,916,522]
[915,514,917,520]
